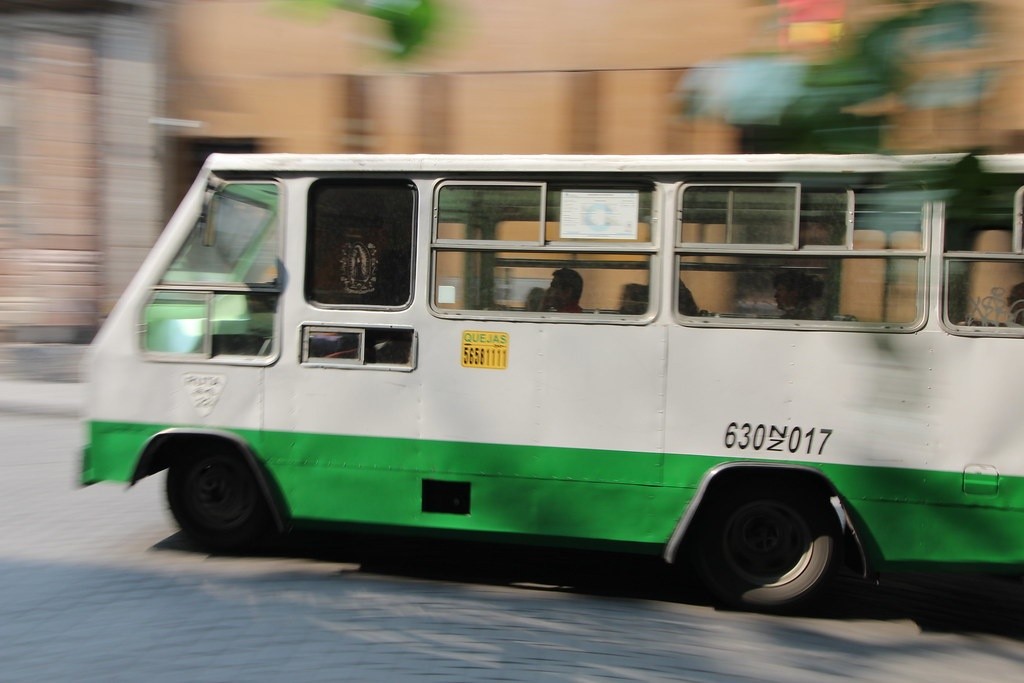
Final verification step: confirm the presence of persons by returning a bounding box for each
[773,268,823,320]
[546,269,585,312]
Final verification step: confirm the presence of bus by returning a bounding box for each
[74,151,1024,616]
